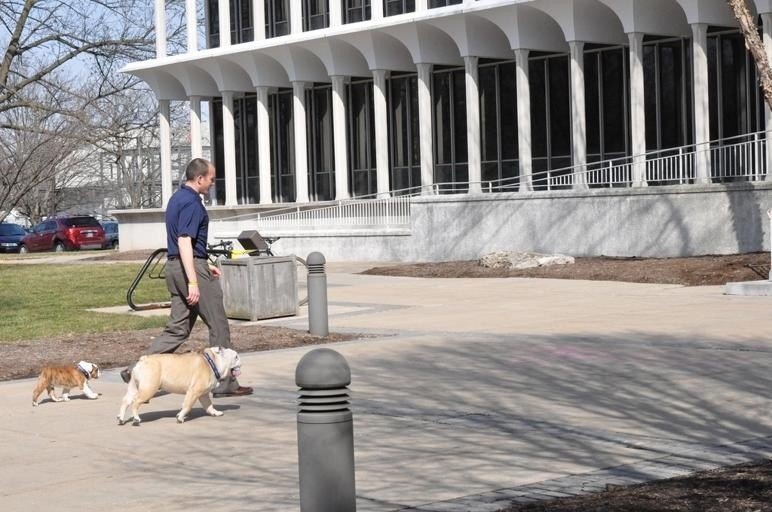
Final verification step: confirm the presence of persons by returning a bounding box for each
[118,157,254,399]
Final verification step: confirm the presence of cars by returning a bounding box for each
[18,216,105,253]
[99,220,119,250]
[0,223,29,253]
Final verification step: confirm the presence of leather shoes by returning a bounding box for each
[121,369,131,382]
[213,384,253,398]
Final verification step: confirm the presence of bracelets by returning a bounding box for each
[188,281,199,286]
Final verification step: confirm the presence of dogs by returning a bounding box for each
[117,344,243,426]
[31,360,101,407]
